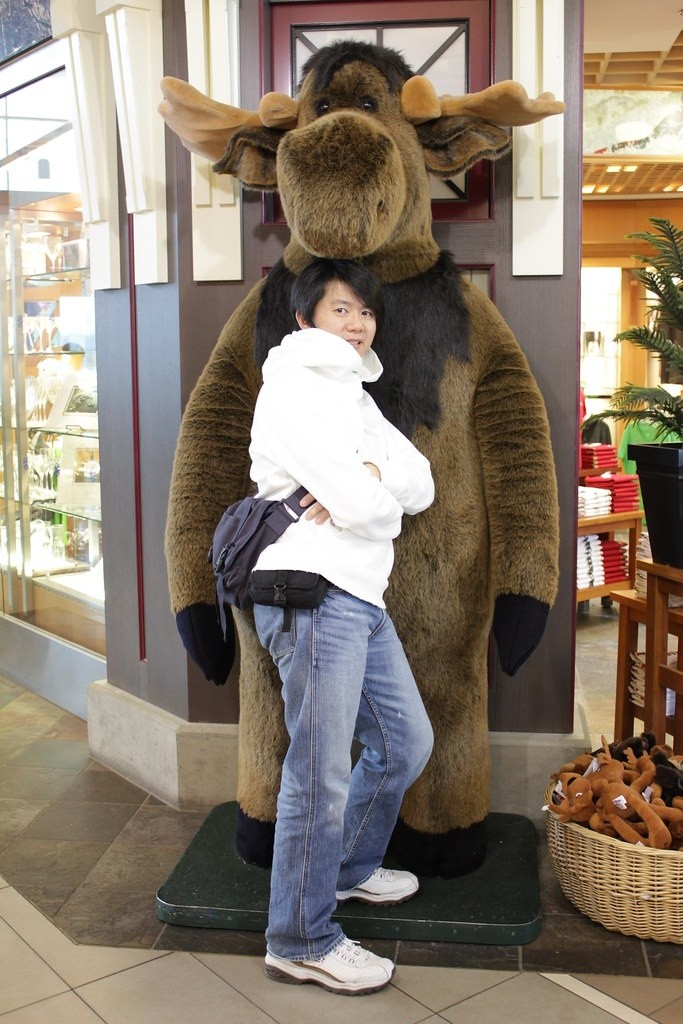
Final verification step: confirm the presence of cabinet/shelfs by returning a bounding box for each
[0,219,108,654]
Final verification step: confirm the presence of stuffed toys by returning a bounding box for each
[541,732,683,852]
[158,40,569,880]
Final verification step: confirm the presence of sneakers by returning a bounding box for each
[263,933,394,995]
[334,866,420,906]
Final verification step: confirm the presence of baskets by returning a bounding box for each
[544,776,683,945]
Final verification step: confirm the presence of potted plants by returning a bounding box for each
[577,218,683,569]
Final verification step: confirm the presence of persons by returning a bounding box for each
[248,257,435,996]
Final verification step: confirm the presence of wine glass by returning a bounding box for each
[28,377,60,424]
[45,236,62,271]
[24,316,58,352]
[28,448,56,501]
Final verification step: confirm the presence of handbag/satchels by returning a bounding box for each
[249,570,332,610]
[207,497,285,611]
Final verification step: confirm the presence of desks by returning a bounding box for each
[577,469,644,602]
[610,591,682,756]
[635,557,683,749]
